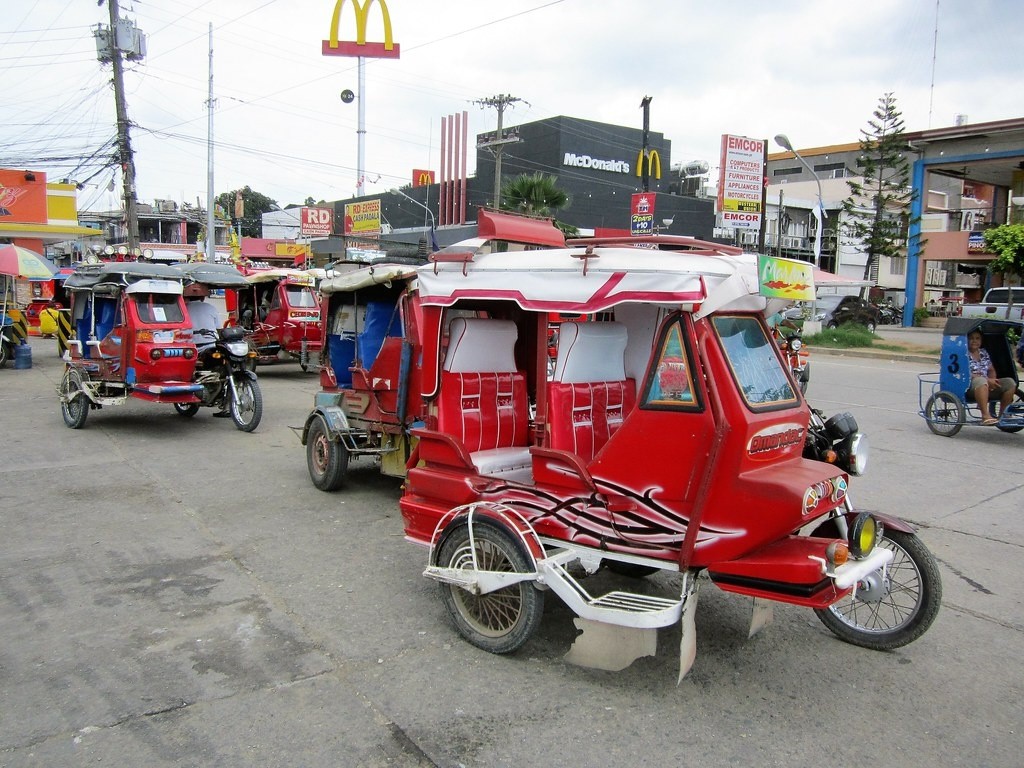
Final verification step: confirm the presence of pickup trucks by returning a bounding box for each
[958,287,1024,323]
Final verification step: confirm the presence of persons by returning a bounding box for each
[766,312,803,339]
[39,304,60,339]
[184,284,223,361]
[239,290,271,329]
[968,330,1017,426]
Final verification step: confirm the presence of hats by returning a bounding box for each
[184,283,210,296]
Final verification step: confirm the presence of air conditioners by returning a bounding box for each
[823,229,833,235]
[159,201,175,212]
[741,232,754,244]
[792,238,802,247]
[823,243,833,249]
[781,237,791,246]
[380,224,390,233]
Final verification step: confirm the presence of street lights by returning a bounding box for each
[774,134,822,269]
[270,204,302,240]
[391,186,435,249]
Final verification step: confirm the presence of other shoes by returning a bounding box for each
[51,334,58,339]
[42,333,46,339]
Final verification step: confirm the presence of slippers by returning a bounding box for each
[979,419,998,426]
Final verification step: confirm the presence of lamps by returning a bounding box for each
[24,170,35,181]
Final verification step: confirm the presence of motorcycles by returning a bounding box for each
[236,268,321,368]
[286,242,454,492]
[57,261,264,433]
[399,208,945,656]
[769,324,810,398]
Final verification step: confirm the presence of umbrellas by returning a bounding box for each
[0,242,60,326]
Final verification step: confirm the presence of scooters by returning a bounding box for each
[875,301,904,325]
[0,326,15,367]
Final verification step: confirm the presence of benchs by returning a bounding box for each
[327,301,402,390]
[531,321,637,494]
[430,318,535,486]
[73,297,123,360]
[965,393,1005,402]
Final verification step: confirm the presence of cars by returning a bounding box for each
[781,294,880,334]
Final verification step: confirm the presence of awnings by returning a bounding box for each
[0,224,103,239]
[142,249,232,261]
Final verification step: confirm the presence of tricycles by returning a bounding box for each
[917,315,1024,434]
